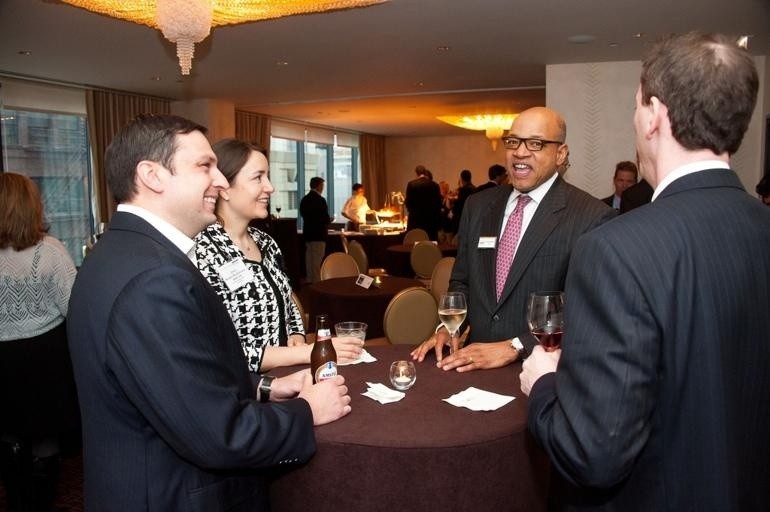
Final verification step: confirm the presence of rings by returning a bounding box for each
[467,355,474,363]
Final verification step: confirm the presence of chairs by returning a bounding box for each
[383,287,438,345]
[410,240,442,279]
[320,252,360,281]
[403,229,429,248]
[348,240,368,275]
[430,257,455,302]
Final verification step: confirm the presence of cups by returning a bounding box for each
[389,360,416,391]
[437,292,467,354]
[334,322,368,361]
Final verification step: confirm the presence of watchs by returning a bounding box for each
[259,372,276,405]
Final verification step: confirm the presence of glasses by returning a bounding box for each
[502,136,562,151]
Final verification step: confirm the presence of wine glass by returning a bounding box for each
[96,222,109,240]
[275,204,282,219]
[524,291,566,352]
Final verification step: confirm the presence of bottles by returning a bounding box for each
[310,316,338,386]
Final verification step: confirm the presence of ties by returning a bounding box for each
[495,195,532,304]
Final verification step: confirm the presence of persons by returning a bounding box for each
[410,106,619,373]
[753,175,770,208]
[404,160,511,241]
[186,134,367,373]
[0,171,78,512]
[518,27,769,511]
[67,111,352,511]
[340,184,376,232]
[599,161,638,214]
[299,176,334,284]
[620,178,652,215]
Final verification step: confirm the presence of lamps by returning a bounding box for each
[63,1,387,76]
[435,113,520,151]
